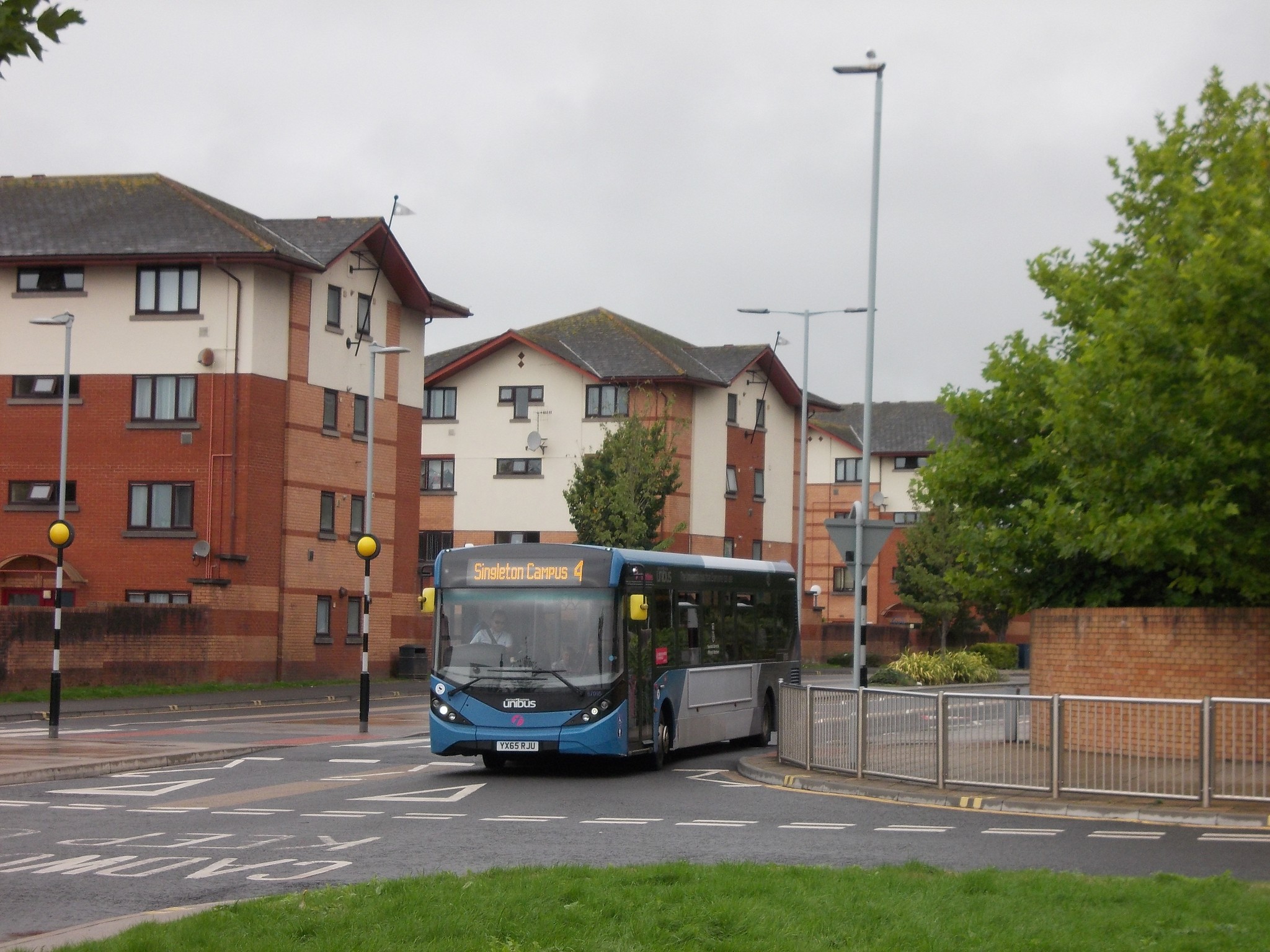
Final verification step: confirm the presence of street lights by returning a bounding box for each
[832,58,888,691]
[737,307,878,636]
[359,341,412,733]
[28,311,75,739]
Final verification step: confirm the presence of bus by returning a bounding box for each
[417,542,802,775]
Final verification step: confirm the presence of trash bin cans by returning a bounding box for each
[1016,643,1030,670]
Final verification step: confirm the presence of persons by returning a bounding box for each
[550,642,599,676]
[470,610,514,648]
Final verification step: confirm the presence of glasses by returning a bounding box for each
[495,620,506,626]
